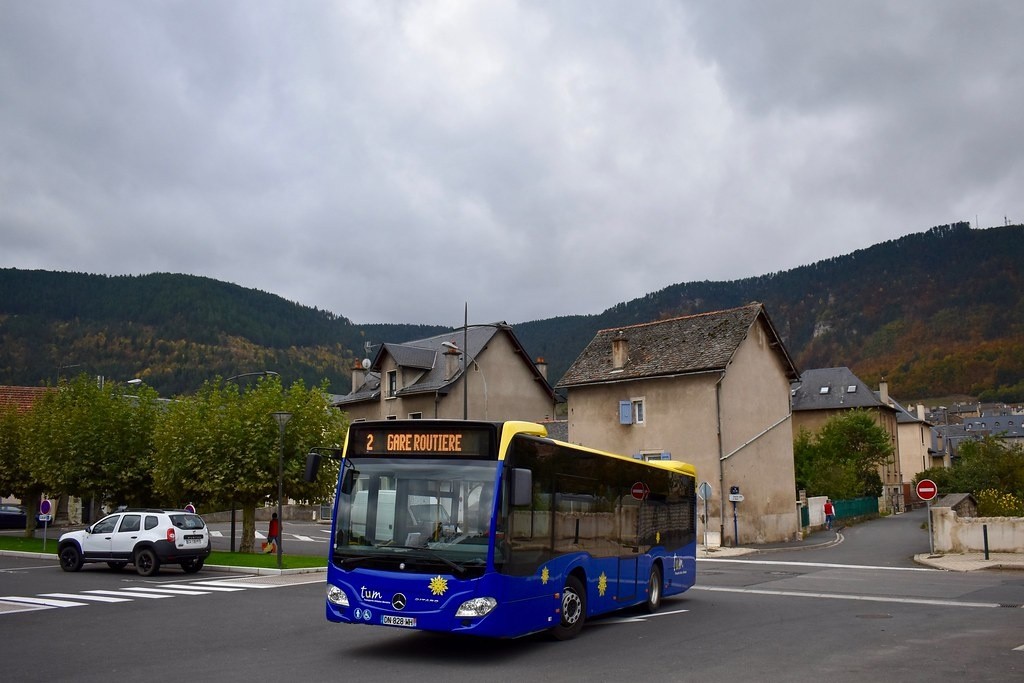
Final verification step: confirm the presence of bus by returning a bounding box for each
[302,420,697,640]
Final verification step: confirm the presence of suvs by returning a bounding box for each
[57,508,211,577]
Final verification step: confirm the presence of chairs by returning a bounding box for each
[409,504,451,526]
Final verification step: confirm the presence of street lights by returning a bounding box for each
[453,301,513,420]
[89,376,141,525]
[226,371,278,552]
[441,342,487,420]
[271,410,293,566]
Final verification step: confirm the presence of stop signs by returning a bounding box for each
[916,479,937,500]
[631,482,650,501]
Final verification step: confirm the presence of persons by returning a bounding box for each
[823,499,836,531]
[266,512,283,553]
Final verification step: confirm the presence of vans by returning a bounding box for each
[349,489,463,545]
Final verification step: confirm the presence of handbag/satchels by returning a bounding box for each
[261,540,276,554]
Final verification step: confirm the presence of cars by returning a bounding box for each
[0,503,53,529]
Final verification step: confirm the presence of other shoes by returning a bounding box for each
[830,528,833,530]
[826,526,828,531]
